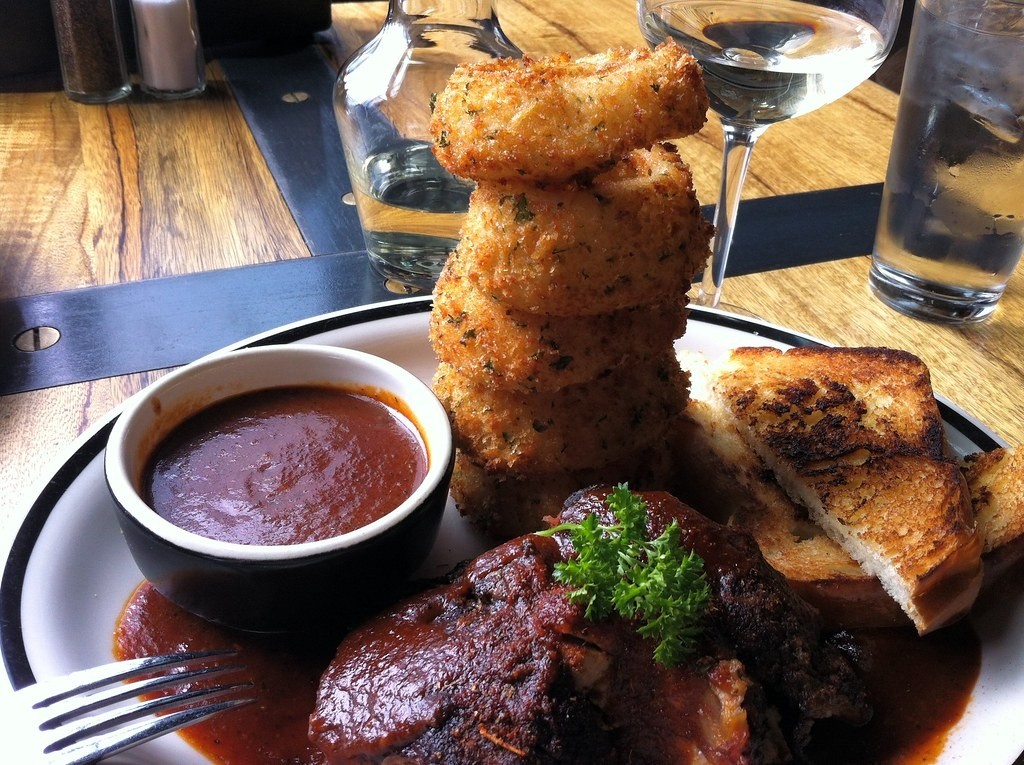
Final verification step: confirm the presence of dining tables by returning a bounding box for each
[0,1,1023,573]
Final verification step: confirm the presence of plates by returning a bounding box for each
[0,289,1024,765]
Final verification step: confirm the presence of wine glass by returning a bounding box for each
[637,0,905,326]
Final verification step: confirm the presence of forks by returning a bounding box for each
[0,645,260,765]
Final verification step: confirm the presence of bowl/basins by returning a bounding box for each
[105,345,456,636]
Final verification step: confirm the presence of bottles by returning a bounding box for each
[331,0,534,290]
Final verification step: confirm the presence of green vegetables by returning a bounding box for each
[534,482,718,675]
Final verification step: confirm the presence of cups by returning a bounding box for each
[130,0,207,100]
[866,0,1024,325]
[50,0,130,105]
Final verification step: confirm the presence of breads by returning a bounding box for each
[681,343,1024,636]
